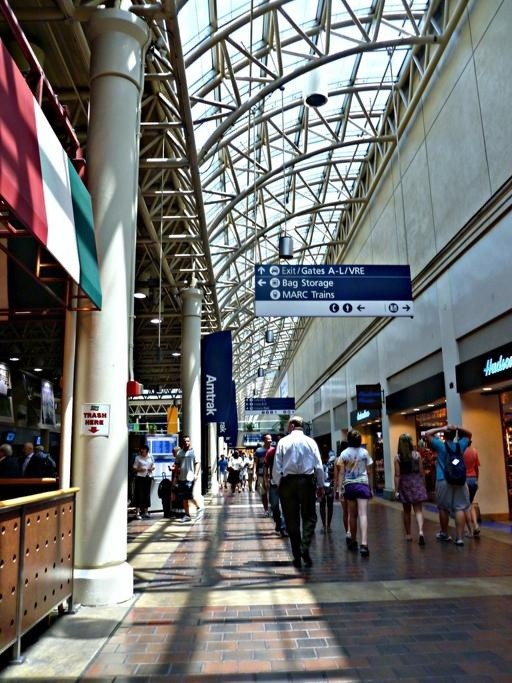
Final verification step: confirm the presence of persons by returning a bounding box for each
[22,445,57,494]
[20,440,34,496]
[0,444,19,499]
[458,435,480,538]
[217,433,337,536]
[338,430,374,558]
[425,425,473,546]
[168,446,181,485]
[132,445,155,519]
[173,435,203,523]
[338,441,358,546]
[270,416,324,569]
[392,433,429,545]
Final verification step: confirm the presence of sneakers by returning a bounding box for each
[293,559,301,568]
[181,516,190,522]
[406,527,480,546]
[321,526,331,533]
[346,532,358,551]
[196,508,203,518]
[300,548,312,564]
[136,511,149,520]
[360,544,369,556]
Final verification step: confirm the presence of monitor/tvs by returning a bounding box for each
[3,431,17,443]
[150,438,178,455]
[34,436,41,445]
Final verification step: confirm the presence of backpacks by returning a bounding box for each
[444,441,466,485]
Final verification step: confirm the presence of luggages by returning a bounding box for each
[159,472,175,518]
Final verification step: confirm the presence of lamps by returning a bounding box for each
[134,265,183,360]
[264,328,281,345]
[251,365,268,399]
[279,235,297,261]
[302,59,329,112]
